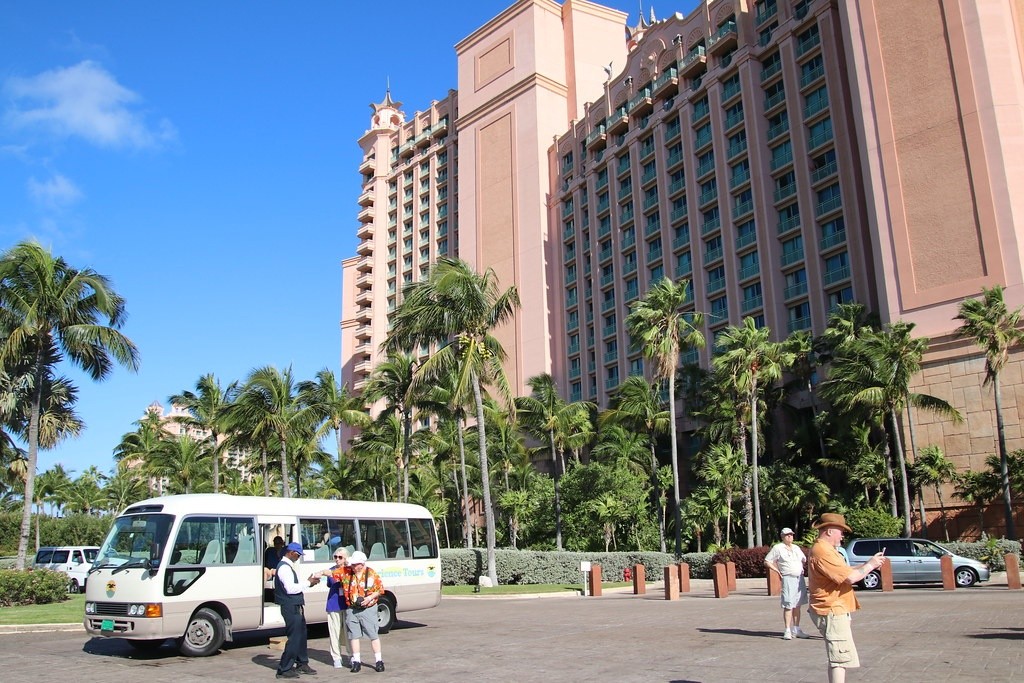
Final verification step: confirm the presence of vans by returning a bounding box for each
[835,537,990,591]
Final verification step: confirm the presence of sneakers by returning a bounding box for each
[791,629,810,639]
[349,656,355,665]
[351,661,361,672]
[276,669,299,679]
[333,660,342,668]
[296,662,317,675]
[783,630,792,640]
[376,661,384,672]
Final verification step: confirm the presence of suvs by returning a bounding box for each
[34,546,129,594]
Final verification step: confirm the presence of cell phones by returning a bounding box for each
[881,547,886,557]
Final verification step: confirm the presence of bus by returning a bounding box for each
[83,493,443,656]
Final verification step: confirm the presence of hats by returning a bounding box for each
[780,527,795,536]
[273,536,284,544]
[812,513,853,533]
[349,551,367,564]
[287,542,305,555]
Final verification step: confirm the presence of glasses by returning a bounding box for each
[294,551,300,557]
[334,554,346,559]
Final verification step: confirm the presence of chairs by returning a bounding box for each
[233,535,255,563]
[200,540,220,564]
[314,542,431,560]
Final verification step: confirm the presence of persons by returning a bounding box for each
[265,536,287,575]
[274,542,320,678]
[314,551,385,673]
[806,514,886,683]
[324,547,353,669]
[764,528,809,640]
